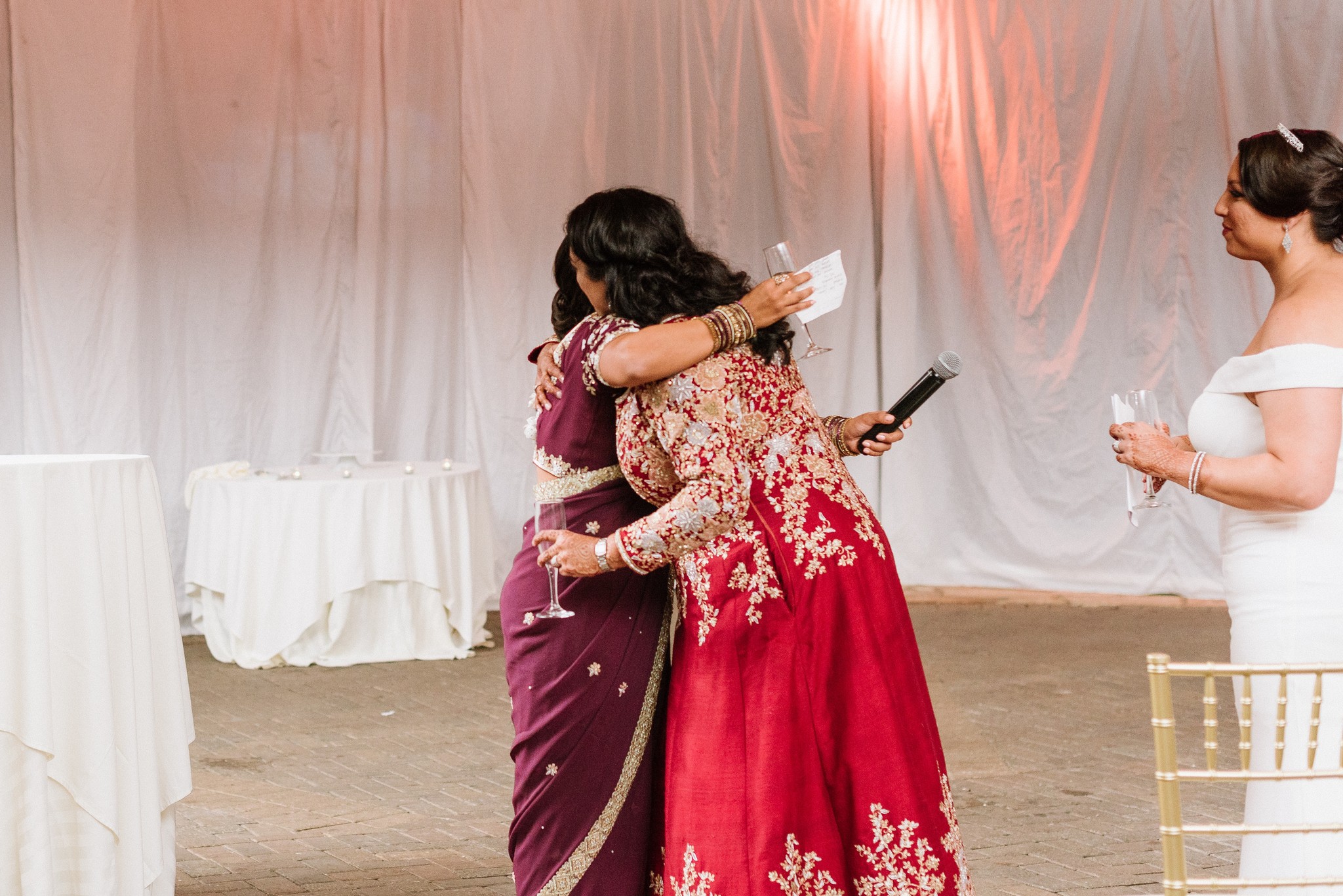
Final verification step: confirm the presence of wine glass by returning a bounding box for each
[1123,390,1171,510]
[763,241,834,360]
[534,500,575,619]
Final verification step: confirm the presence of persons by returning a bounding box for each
[1109,123,1343,894]
[531,185,973,896]
[500,230,913,896]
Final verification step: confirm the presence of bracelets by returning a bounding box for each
[1188,451,1206,495]
[821,414,860,458]
[691,299,757,356]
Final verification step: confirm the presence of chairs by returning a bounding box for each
[1145,650,1343,896]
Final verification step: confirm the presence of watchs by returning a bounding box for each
[593,537,616,572]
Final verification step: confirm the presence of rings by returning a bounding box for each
[533,383,542,391]
[1112,440,1123,453]
[771,273,790,286]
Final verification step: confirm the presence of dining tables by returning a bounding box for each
[185,456,495,673]
[0,452,195,896]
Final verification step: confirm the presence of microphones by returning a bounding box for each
[854,350,963,454]
